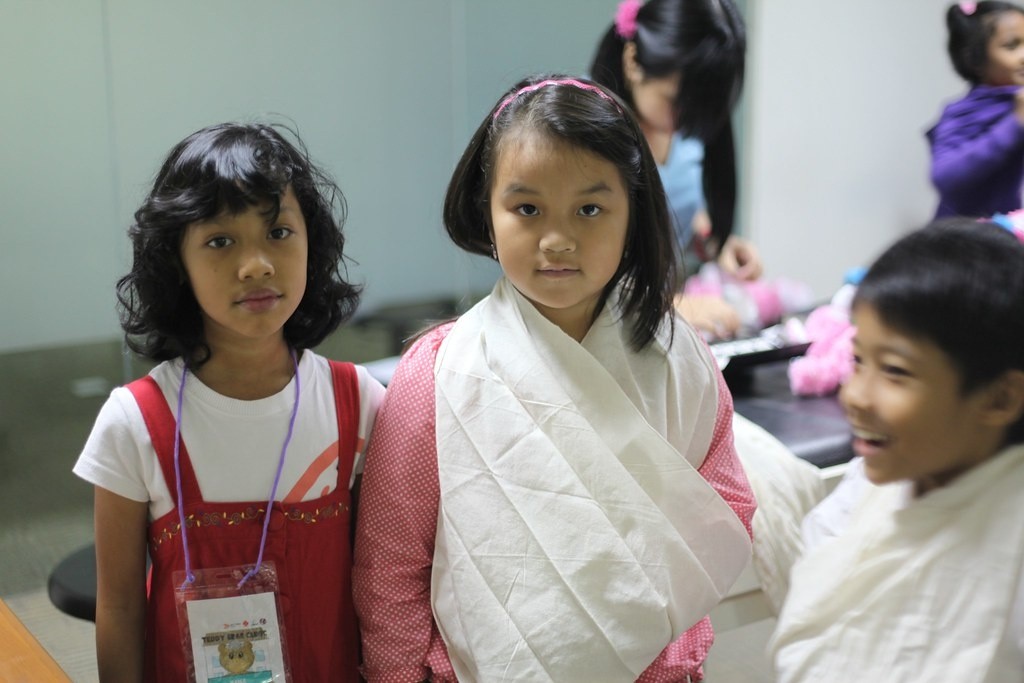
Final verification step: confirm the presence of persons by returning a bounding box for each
[72,121,388,683]
[588,0,763,297]
[766,220,1024,683]
[923,0,1024,223]
[351,73,759,683]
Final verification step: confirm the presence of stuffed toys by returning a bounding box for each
[991,208,1024,243]
[784,266,872,394]
[666,259,782,344]
